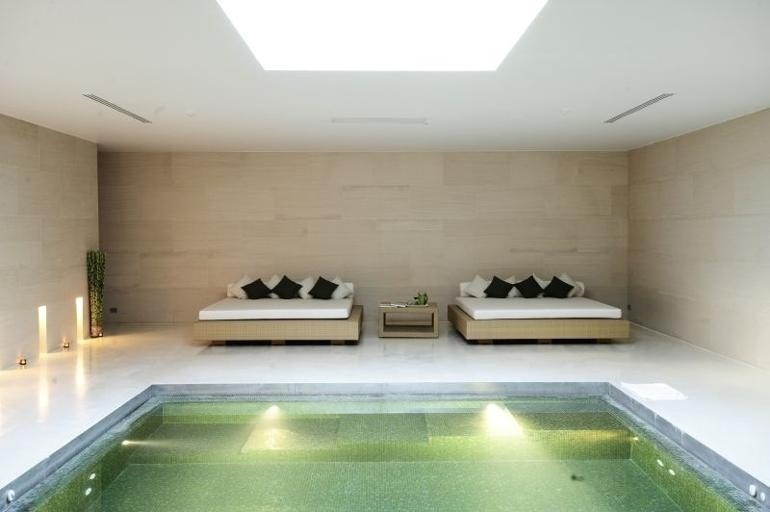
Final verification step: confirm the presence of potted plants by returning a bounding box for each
[87,249,106,338]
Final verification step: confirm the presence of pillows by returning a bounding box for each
[464,273,580,298]
[230,272,353,300]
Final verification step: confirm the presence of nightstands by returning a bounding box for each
[379,302,439,338]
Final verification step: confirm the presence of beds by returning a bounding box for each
[194,283,361,345]
[449,280,630,344]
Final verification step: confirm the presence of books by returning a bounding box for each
[395,301,409,308]
[378,301,397,309]
